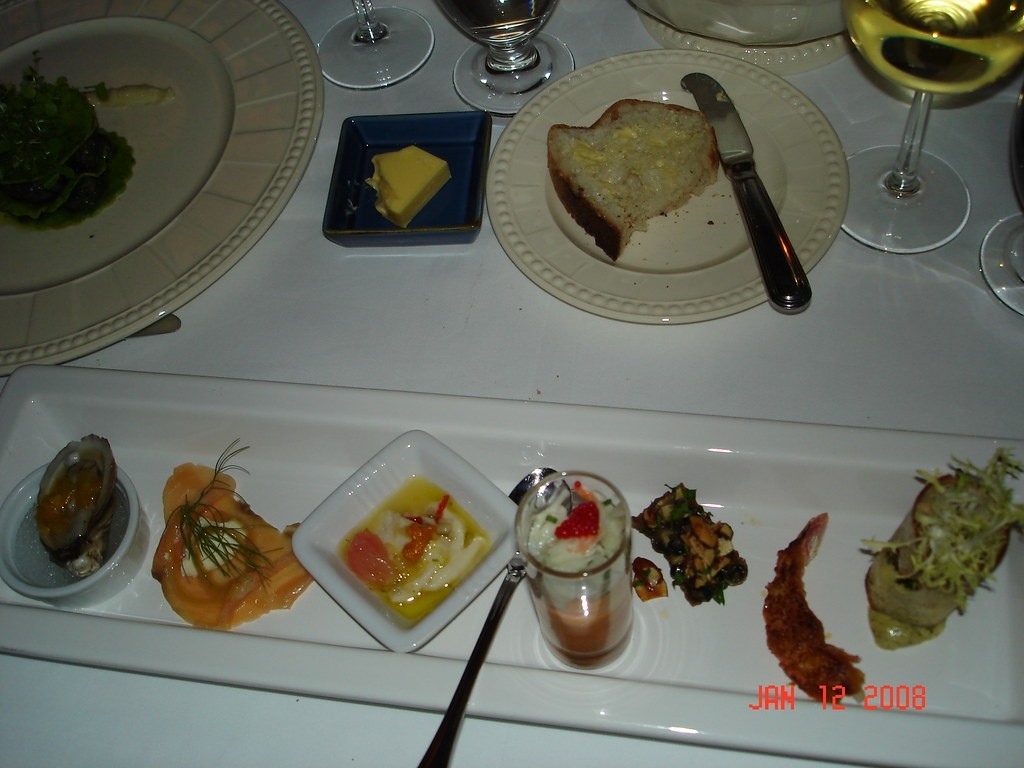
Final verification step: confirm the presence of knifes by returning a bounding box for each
[683,72,813,314]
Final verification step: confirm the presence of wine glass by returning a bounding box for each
[433,0,576,115]
[838,0,1024,253]
[978,78,1024,319]
[317,0,435,90]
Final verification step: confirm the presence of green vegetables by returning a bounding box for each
[632,486,730,606]
[179,437,276,592]
[0,50,133,227]
[860,444,1024,615]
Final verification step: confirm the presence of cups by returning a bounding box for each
[514,472,636,665]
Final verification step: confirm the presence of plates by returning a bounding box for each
[625,0,854,72]
[320,109,492,245]
[0,0,324,372]
[485,48,849,323]
[292,428,526,653]
[1,361,1024,768]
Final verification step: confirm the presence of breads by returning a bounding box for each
[547,98,718,263]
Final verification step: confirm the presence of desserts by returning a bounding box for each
[367,146,451,228]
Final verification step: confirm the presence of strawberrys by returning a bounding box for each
[555,503,600,551]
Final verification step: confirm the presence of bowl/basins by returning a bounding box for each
[1,462,150,609]
